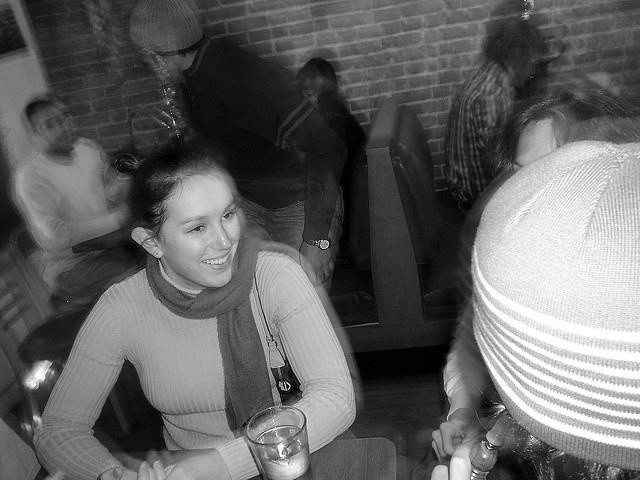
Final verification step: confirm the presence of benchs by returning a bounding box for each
[328,96,465,354]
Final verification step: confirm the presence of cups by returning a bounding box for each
[245,406,311,479]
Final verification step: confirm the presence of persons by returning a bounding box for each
[297,59,367,194]
[32,140,356,480]
[444,16,547,225]
[129,0,344,291]
[430,142,640,480]
[429,78,640,461]
[12,97,148,302]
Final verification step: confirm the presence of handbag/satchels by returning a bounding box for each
[267,346,302,407]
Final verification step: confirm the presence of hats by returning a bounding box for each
[127,0,206,54]
[466,140,640,472]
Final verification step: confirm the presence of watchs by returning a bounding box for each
[303,239,332,250]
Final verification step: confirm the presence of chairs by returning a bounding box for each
[1,226,137,439]
